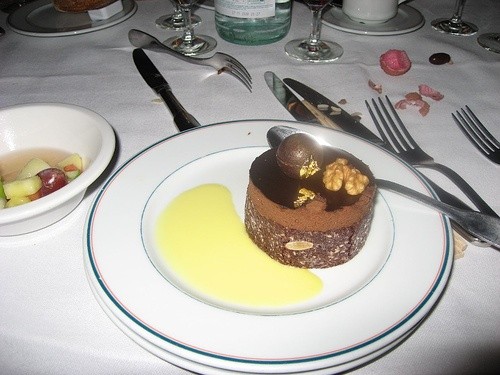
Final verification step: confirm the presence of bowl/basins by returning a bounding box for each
[0,102,116,237]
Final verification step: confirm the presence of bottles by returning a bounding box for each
[214,0,291,46]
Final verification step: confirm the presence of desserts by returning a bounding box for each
[244,133,377,268]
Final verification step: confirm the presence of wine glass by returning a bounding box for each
[476,32,500,53]
[285,0,344,64]
[155,0,201,32]
[170,0,209,56]
[431,0,479,37]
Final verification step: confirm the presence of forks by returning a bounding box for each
[128,29,253,93]
[450,105,500,165]
[365,95,500,219]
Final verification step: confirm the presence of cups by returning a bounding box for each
[342,0,406,25]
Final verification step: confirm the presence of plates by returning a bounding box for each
[320,3,425,36]
[6,0,138,37]
[81,119,455,375]
[194,0,215,10]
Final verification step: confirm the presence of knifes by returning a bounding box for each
[264,71,491,247]
[132,48,201,132]
[283,78,474,212]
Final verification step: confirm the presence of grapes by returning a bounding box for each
[33,169,66,197]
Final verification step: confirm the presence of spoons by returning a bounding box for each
[266,125,500,249]
